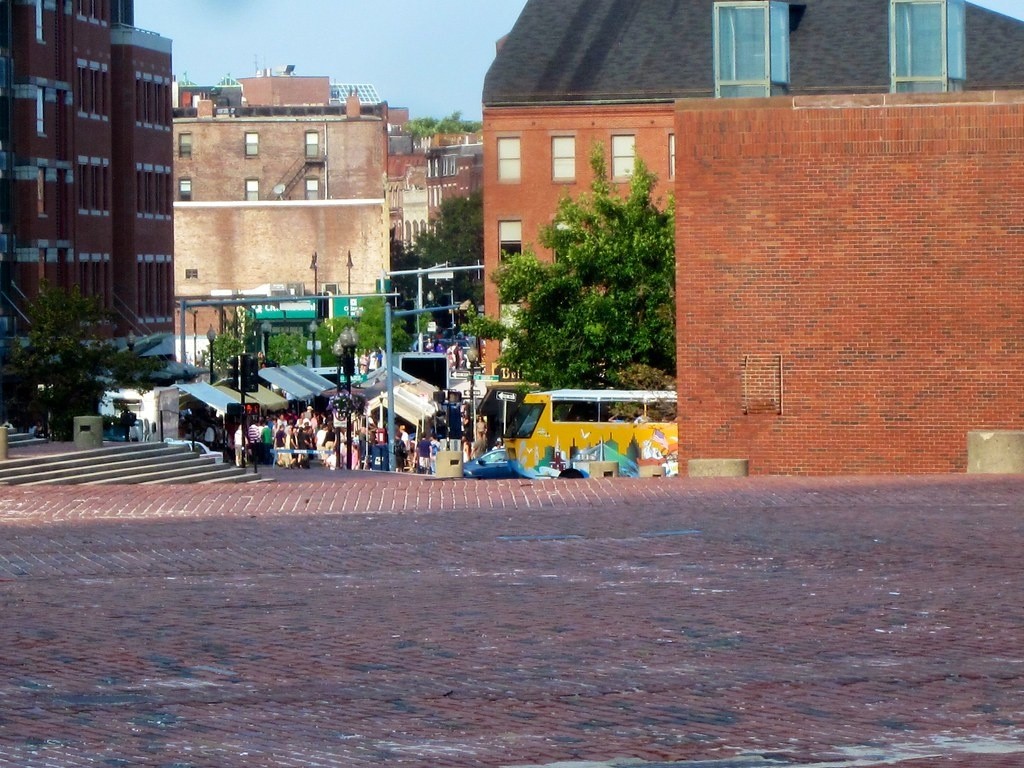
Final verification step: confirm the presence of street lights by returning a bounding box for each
[340,325,359,469]
[160,409,185,442]
[310,254,318,295]
[309,320,318,367]
[207,323,216,419]
[346,250,353,295]
[354,307,362,321]
[261,318,272,368]
[428,291,434,350]
[126,330,136,353]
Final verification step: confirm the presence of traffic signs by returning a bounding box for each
[464,390,480,395]
[496,390,517,402]
[449,370,469,380]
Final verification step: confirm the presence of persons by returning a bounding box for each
[261,363,265,368]
[559,406,582,421]
[180,406,378,470]
[281,390,315,410]
[608,405,677,424]
[196,350,202,365]
[258,352,263,358]
[32,420,52,438]
[476,416,487,442]
[413,336,465,374]
[121,408,135,440]
[462,414,478,463]
[359,346,387,375]
[491,441,501,462]
[395,425,441,474]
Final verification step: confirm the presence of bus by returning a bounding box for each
[503,389,677,479]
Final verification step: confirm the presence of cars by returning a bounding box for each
[412,338,470,352]
[164,439,223,461]
[461,448,517,480]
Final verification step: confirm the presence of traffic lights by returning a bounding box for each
[449,392,463,402]
[228,356,239,388]
[246,402,259,415]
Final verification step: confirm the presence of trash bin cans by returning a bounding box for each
[371,443,389,470]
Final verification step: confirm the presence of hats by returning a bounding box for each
[399,425,406,430]
[307,406,314,410]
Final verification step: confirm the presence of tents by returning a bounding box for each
[170,377,288,460]
[282,364,338,396]
[366,380,444,467]
[251,367,320,417]
